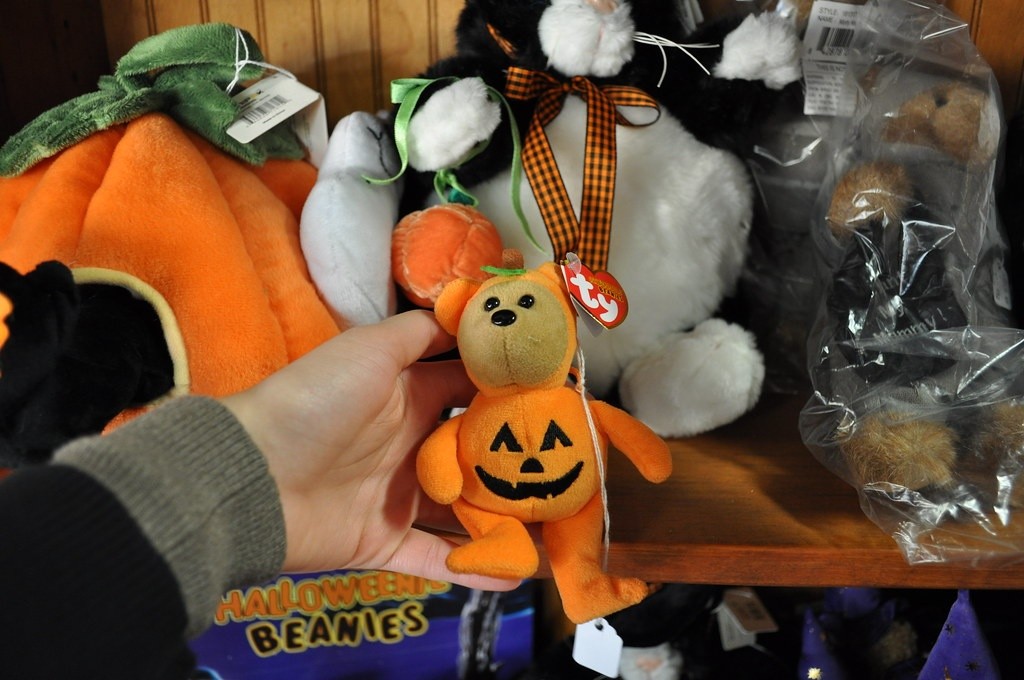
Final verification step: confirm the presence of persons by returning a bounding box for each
[2,308,530,680]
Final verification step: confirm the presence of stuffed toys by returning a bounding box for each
[392,0,808,438]
[414,251,674,622]
[817,82,1024,495]
[0,21,396,478]
